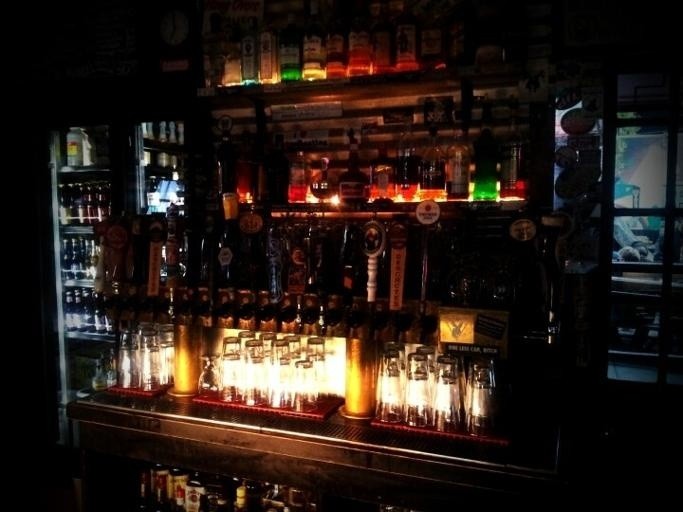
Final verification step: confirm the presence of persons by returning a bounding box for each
[613,216,683,262]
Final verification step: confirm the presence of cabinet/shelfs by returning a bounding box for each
[185,65,554,418]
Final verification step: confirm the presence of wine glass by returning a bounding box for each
[199,354,222,396]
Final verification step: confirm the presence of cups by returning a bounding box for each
[376,343,500,438]
[441,265,517,310]
[120,321,175,390]
[223,330,326,409]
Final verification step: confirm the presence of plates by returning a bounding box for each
[167,386,198,397]
[339,403,373,420]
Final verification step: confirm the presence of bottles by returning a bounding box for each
[113,286,432,341]
[346,337,372,416]
[139,459,322,511]
[174,325,198,394]
[146,176,160,208]
[57,181,110,226]
[284,95,525,205]
[64,287,112,331]
[161,176,171,211]
[141,122,183,174]
[62,236,104,282]
[222,10,450,84]
[91,358,107,392]
[67,127,84,166]
